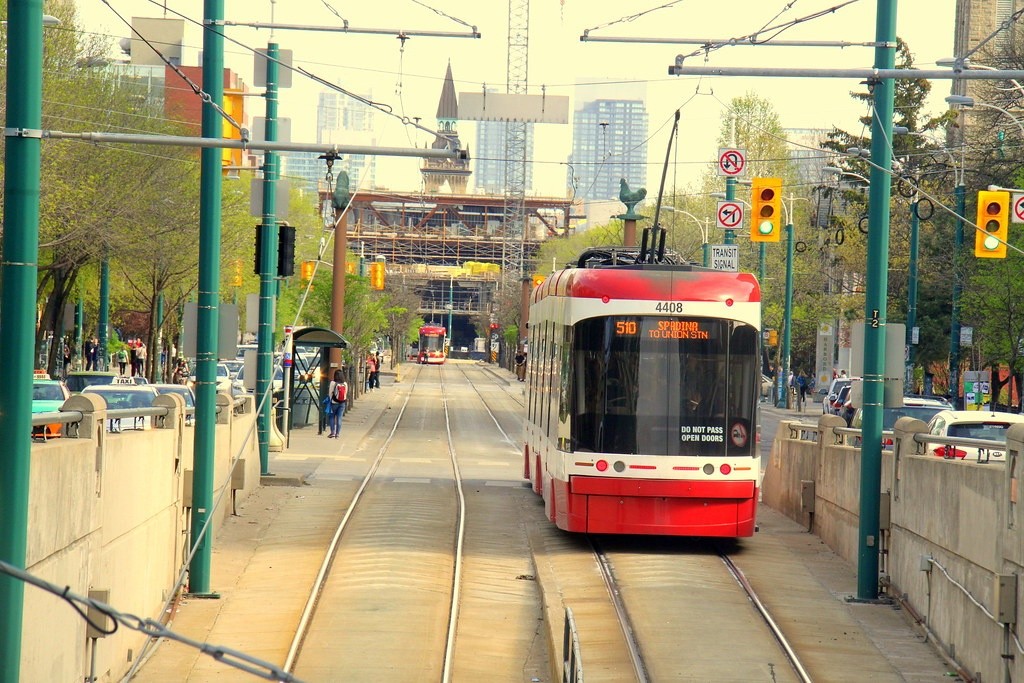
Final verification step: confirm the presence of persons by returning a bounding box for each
[64,333,189,384]
[515,350,527,382]
[423,346,430,364]
[768,364,848,402]
[327,369,348,438]
[366,351,381,389]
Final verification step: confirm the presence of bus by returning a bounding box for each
[417,324,448,361]
[527,272,769,538]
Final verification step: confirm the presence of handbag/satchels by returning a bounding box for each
[376,363,380,370]
[804,385,809,390]
[132,356,139,364]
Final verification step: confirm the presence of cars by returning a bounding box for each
[220,340,320,386]
[822,377,863,431]
[851,393,962,454]
[71,376,157,437]
[233,360,284,399]
[68,370,122,396]
[920,410,1024,463]
[184,364,233,392]
[32,370,69,430]
[144,383,194,430]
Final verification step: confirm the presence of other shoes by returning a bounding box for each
[366,386,380,393]
[517,378,524,382]
[328,434,339,439]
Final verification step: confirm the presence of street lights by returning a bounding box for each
[823,162,870,187]
[844,143,922,392]
[889,126,968,401]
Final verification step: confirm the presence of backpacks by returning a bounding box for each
[332,382,346,403]
[118,350,125,359]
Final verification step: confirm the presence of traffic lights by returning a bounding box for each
[751,179,782,245]
[976,189,1009,258]
